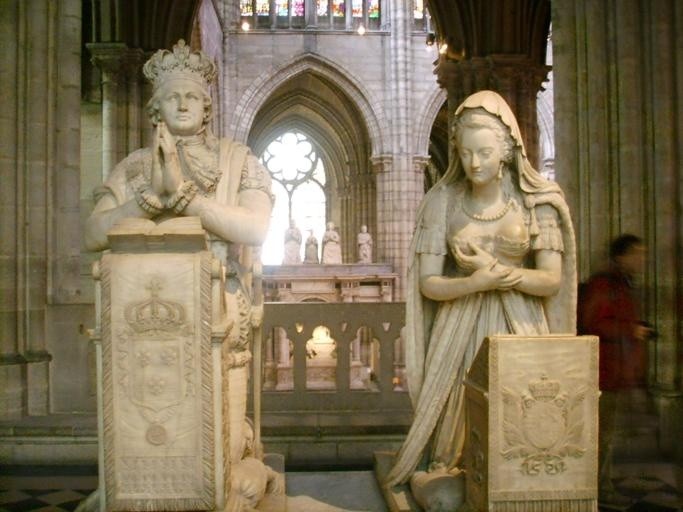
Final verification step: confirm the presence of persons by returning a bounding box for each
[383,89,580,510]
[321,221,342,264]
[278,218,300,266]
[354,221,375,263]
[302,226,319,265]
[80,37,263,463]
[579,233,665,500]
